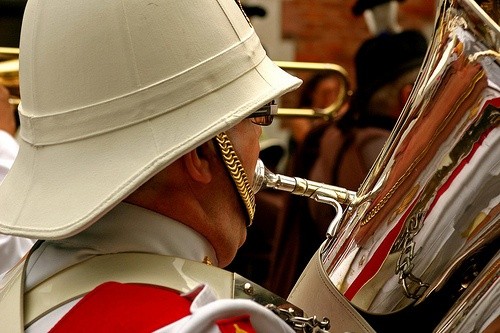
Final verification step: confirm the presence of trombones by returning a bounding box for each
[1,45,351,122]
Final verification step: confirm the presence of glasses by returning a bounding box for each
[246,100,276,126]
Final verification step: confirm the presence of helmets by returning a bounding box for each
[0,0,302,242]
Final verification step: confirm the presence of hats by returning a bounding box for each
[349,27,427,97]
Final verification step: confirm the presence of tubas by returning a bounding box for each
[250,0,500,333]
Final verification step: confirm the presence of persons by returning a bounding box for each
[288,69,345,177]
[2,0,305,333]
[286,27,430,248]
